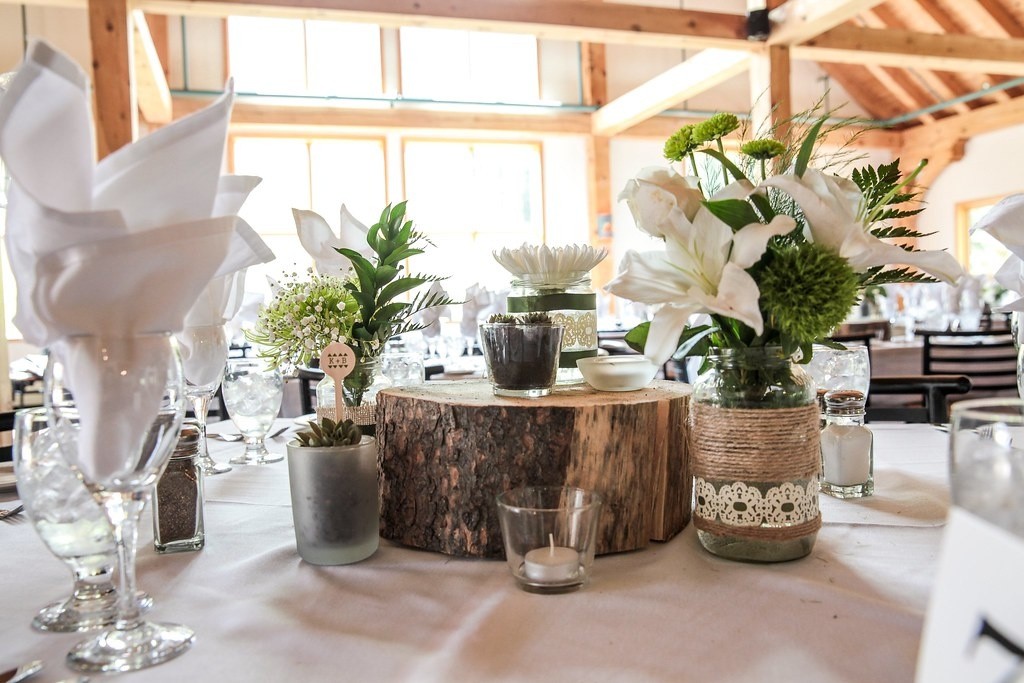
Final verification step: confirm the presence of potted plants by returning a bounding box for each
[286,417,380,567]
[478,312,565,399]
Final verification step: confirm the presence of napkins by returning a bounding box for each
[0,41,277,482]
[293,204,379,279]
[967,192,1024,313]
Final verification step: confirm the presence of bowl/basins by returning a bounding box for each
[576,354,659,391]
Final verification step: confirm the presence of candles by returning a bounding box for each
[525,532,580,581]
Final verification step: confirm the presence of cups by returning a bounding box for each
[380,353,425,391]
[948,398,1023,538]
[890,317,914,344]
[497,486,601,594]
[808,345,870,430]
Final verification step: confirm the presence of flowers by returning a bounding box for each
[603,86,962,422]
[489,243,607,294]
[239,200,472,408]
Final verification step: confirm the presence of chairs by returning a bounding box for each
[866,330,1019,427]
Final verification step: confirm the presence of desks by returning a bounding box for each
[0,418,953,683]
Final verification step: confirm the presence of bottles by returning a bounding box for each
[818,390,874,499]
[154,417,206,554]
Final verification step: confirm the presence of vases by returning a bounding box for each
[687,344,822,563]
[315,355,394,438]
[507,272,599,386]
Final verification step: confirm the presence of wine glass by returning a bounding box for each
[221,357,284,465]
[44,331,195,672]
[13,406,154,633]
[169,326,233,475]
